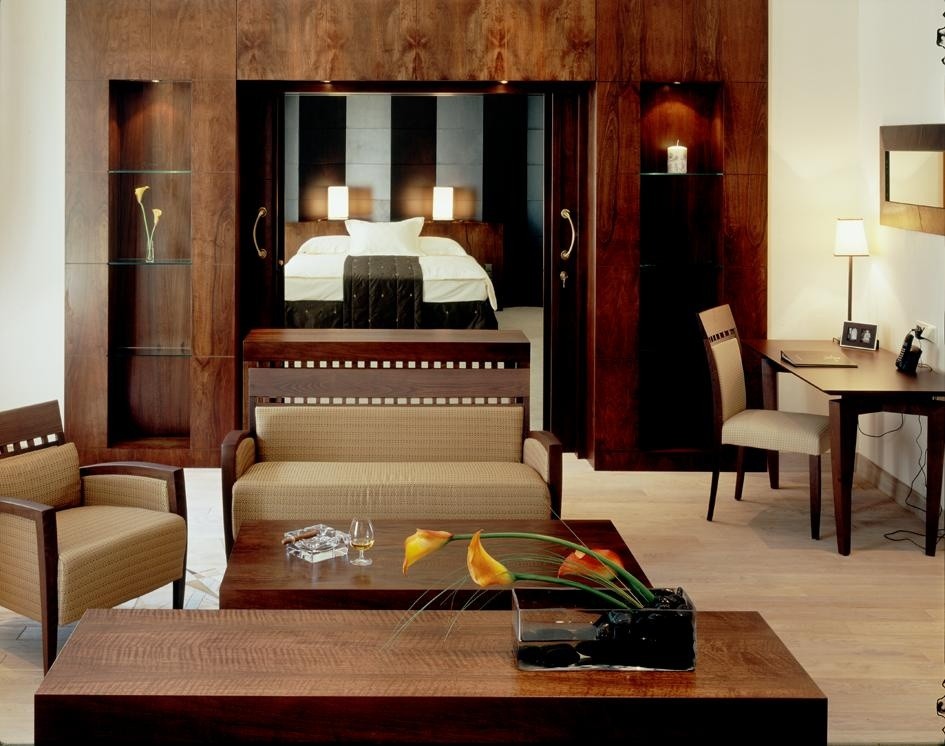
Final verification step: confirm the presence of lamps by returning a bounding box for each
[833,217,870,343]
[431,185,455,222]
[327,186,348,220]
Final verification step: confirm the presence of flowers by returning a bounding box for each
[382,496,653,651]
[134,186,163,259]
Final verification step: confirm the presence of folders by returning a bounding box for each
[780,350,857,368]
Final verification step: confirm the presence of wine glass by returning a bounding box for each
[348,516,374,567]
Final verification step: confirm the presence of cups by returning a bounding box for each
[667,145,687,174]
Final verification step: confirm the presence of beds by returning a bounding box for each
[283,220,505,329]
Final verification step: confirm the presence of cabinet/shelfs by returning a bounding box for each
[642,81,722,450]
[108,80,191,450]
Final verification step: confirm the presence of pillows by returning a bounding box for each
[295,234,351,256]
[343,216,427,258]
[419,237,468,258]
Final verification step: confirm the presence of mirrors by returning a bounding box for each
[879,123,945,236]
[284,91,547,427]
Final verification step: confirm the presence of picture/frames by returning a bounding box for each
[839,321,879,350]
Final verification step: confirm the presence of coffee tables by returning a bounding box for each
[220,520,653,610]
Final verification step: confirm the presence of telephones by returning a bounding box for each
[896,334,922,375]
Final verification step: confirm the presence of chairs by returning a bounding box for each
[697,303,831,541]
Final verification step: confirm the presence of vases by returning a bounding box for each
[145,241,154,263]
[511,586,699,673]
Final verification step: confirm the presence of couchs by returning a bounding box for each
[0,400,189,676]
[220,368,562,562]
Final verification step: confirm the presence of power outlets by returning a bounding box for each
[915,320,937,343]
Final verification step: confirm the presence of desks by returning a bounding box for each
[243,327,532,429]
[737,339,945,557]
[34,608,830,745]
